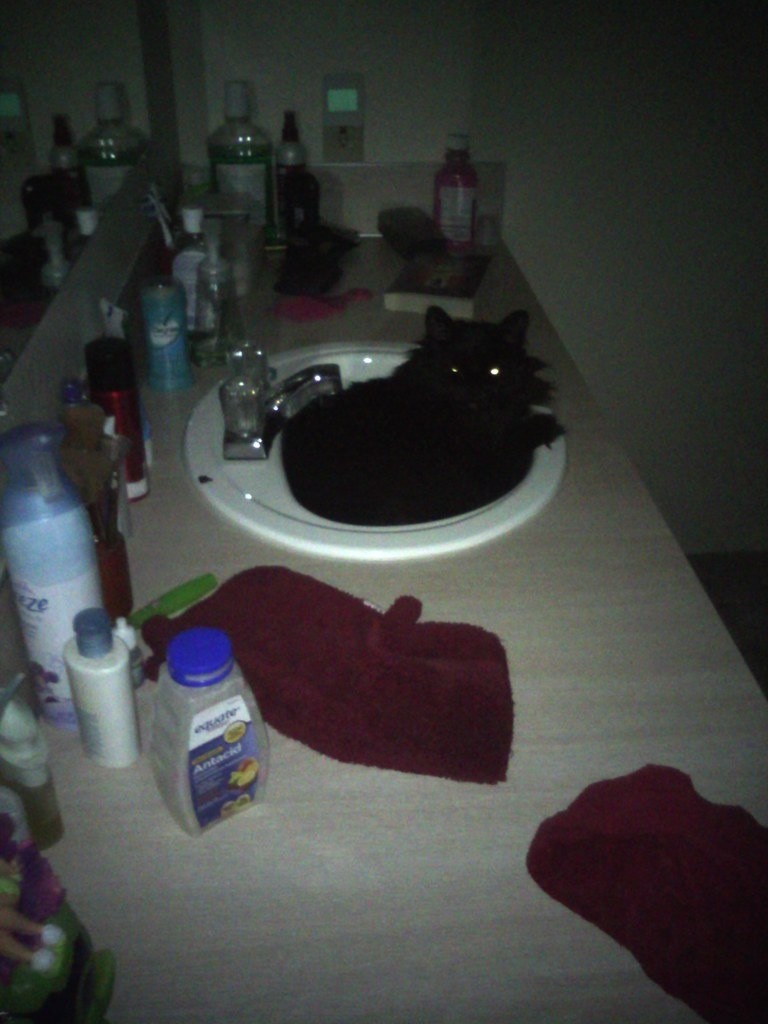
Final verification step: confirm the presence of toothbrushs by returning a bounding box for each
[141,183,176,251]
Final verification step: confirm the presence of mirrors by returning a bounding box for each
[0,0,153,385]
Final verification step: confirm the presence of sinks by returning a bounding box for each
[182,339,569,563]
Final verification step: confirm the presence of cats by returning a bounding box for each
[283,306,565,526]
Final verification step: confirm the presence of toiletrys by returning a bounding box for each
[62,605,142,771]
[2,79,151,362]
[0,698,66,851]
[1,336,153,733]
[433,132,479,253]
[138,260,197,392]
[150,625,269,837]
[172,203,245,360]
[208,80,322,247]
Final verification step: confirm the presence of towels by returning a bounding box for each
[519,756,767,1023]
[138,562,517,784]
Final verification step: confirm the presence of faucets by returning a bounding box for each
[264,363,345,433]
